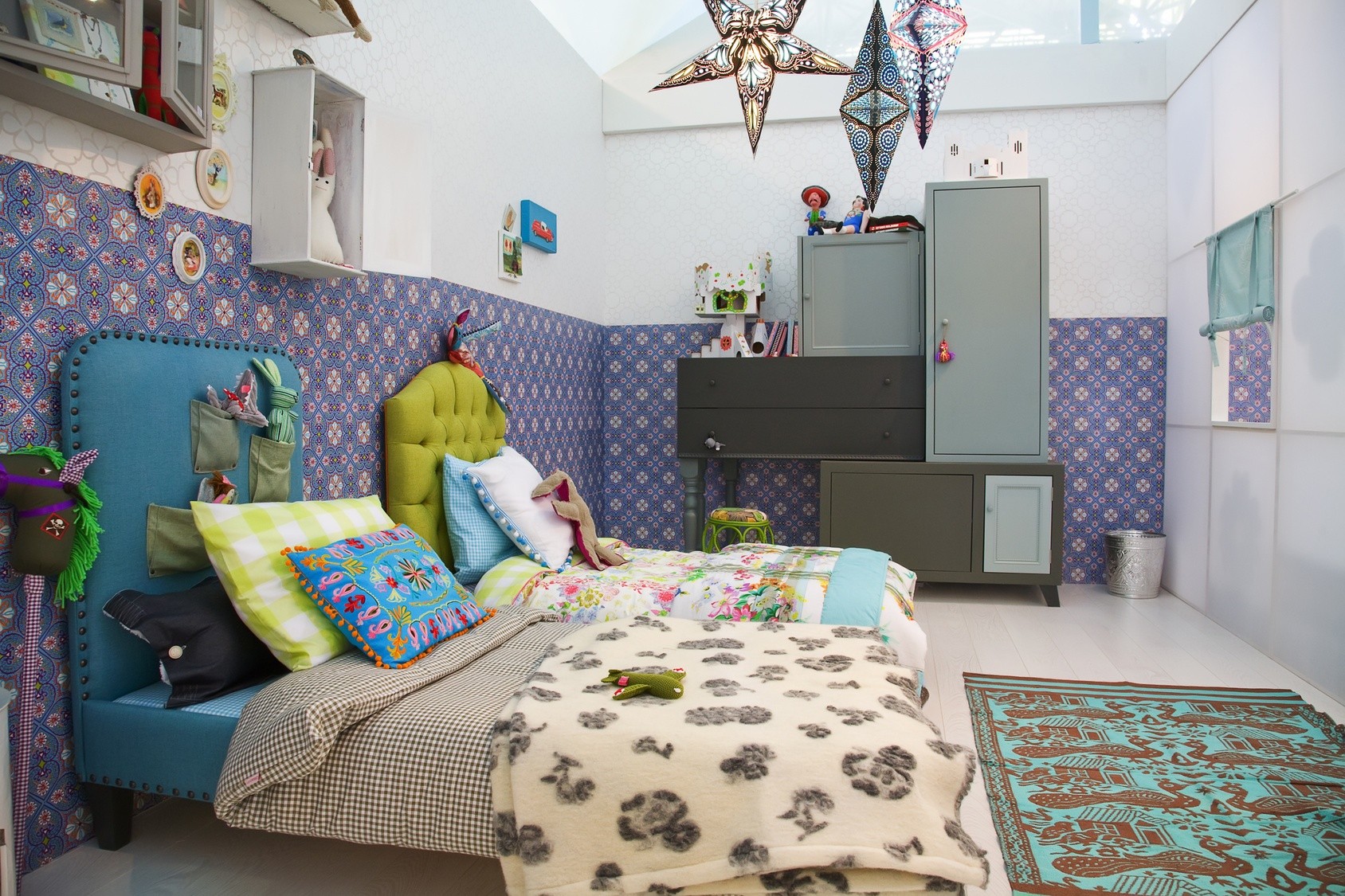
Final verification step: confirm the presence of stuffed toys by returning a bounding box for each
[531,467,628,571]
[602,669,686,699]
[0,445,103,610]
[206,369,267,428]
[311,127,354,268]
[252,356,299,442]
[446,308,512,416]
[130,26,177,125]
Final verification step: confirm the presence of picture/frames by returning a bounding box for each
[212,53,238,133]
[197,146,233,210]
[172,232,206,284]
[134,165,167,222]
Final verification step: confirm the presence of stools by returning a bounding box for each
[702,507,774,553]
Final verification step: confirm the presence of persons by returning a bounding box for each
[832,195,869,234]
[208,471,236,505]
[184,247,196,265]
[146,183,156,208]
[801,185,831,236]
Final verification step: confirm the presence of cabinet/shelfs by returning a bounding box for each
[797,233,924,358]
[251,67,367,281]
[925,178,1049,461]
[675,355,924,462]
[818,462,1064,607]
[0,0,214,154]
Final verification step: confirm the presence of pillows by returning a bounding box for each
[193,494,397,671]
[443,452,521,585]
[102,575,289,710]
[464,446,579,573]
[283,525,495,668]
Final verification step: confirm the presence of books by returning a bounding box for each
[19,0,136,112]
[794,321,799,356]
[762,319,788,357]
[869,221,919,233]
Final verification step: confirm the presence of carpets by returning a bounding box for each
[962,672,1345,896]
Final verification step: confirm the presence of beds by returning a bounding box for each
[386,361,929,707]
[63,330,989,896]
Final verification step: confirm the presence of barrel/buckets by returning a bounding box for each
[1101,530,1167,599]
